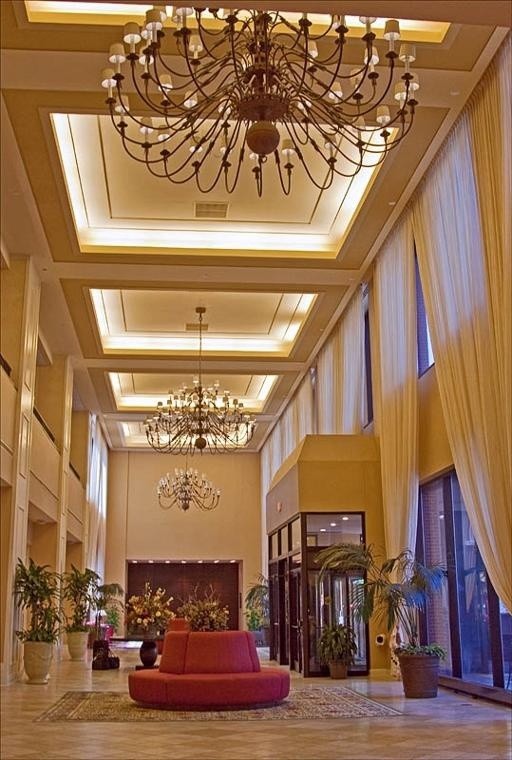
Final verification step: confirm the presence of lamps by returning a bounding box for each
[155,456,222,514]
[138,306,258,458]
[97,5,424,202]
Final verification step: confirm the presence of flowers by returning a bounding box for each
[179,584,234,632]
[126,582,176,640]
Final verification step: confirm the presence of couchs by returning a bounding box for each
[126,630,292,712]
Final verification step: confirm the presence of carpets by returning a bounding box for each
[31,686,415,723]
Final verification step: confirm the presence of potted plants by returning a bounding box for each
[313,540,449,699]
[11,557,123,685]
[244,571,270,647]
[317,622,359,681]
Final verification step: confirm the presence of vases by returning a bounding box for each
[135,631,160,671]
